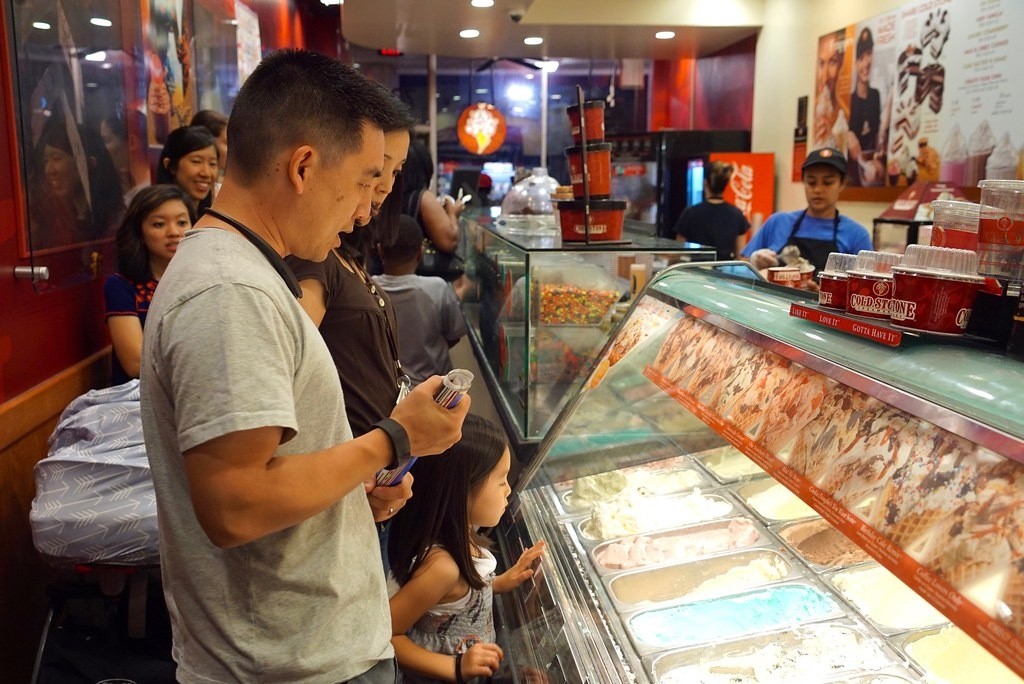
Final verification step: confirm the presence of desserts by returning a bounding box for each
[767,179,1024,338]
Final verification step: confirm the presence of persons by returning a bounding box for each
[743,145,872,293]
[88,45,549,684]
[674,157,751,265]
[23,32,202,249]
[810,24,942,190]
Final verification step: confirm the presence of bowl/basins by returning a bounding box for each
[817,252,858,311]
[769,267,801,290]
[888,244,985,336]
[784,263,817,289]
[845,249,904,321]
[558,198,628,243]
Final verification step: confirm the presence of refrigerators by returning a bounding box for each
[686,151,775,243]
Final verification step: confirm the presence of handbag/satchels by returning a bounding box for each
[410,186,464,282]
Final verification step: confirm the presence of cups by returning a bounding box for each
[976,179,1024,282]
[942,147,1019,189]
[834,128,850,160]
[929,200,979,250]
[565,142,611,199]
[567,99,605,145]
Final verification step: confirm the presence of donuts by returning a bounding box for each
[891,7,950,153]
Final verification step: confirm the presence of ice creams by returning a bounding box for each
[148,28,191,143]
[942,119,1019,186]
[655,314,1024,639]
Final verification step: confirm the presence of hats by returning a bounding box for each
[802,147,849,174]
[856,28,873,58]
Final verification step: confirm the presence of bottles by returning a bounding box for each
[551,185,573,213]
